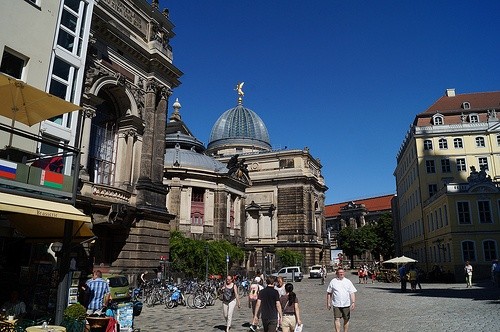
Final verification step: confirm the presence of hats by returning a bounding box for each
[253,276,261,282]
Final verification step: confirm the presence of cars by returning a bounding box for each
[333,264,344,271]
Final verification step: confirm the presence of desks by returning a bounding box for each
[25,325,67,332]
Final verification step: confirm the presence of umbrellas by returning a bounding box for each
[382,255,419,269]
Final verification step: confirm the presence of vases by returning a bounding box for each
[87,316,109,332]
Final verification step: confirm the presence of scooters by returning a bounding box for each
[129,287,143,316]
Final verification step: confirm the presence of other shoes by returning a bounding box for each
[249,325,256,331]
[256,325,262,330]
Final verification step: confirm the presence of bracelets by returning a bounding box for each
[352,302,354,303]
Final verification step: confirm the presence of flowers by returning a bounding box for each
[89,308,107,317]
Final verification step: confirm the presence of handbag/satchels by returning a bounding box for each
[218,294,223,301]
[294,323,303,332]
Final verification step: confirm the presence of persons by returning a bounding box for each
[249,276,264,332]
[464,260,473,288]
[397,264,423,291]
[488,258,500,287]
[274,276,287,331]
[358,262,376,284]
[432,263,442,282]
[472,262,480,283]
[254,277,282,332]
[3,288,27,316]
[86,270,112,332]
[280,283,302,332]
[138,271,148,287]
[219,275,240,332]
[233,272,271,297]
[327,268,355,332]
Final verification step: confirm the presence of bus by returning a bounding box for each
[343,260,352,270]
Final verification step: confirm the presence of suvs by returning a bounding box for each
[270,267,304,282]
[310,265,328,279]
[101,275,132,304]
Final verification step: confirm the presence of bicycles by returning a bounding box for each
[141,279,251,309]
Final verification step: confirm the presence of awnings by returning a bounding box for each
[0,192,92,223]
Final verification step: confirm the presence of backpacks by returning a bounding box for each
[248,284,259,301]
